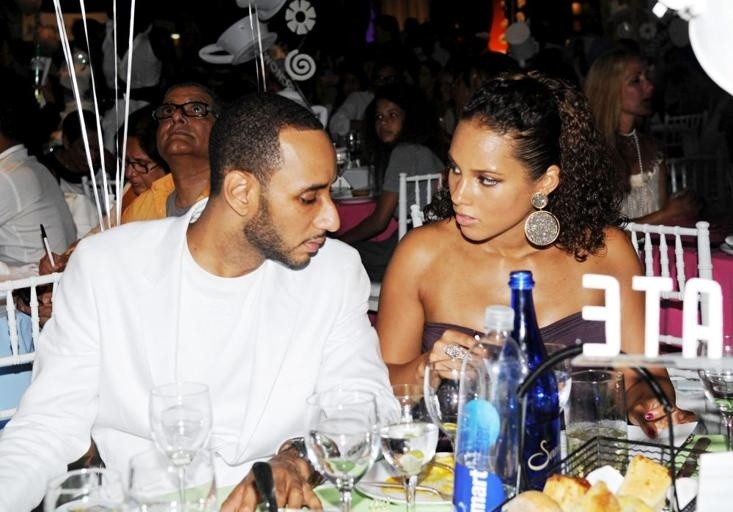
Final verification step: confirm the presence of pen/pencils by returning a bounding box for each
[40,224,55,268]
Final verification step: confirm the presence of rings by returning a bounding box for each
[441,346,475,362]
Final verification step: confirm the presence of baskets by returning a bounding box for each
[492,435,711,512]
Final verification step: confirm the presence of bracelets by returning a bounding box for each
[283,440,323,485]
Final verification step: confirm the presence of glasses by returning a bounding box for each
[118,157,157,173]
[152,101,218,120]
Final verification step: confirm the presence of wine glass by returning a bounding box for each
[306,391,381,510]
[375,384,442,510]
[149,383,214,508]
[697,370,733,451]
[333,142,351,194]
[670,159,697,194]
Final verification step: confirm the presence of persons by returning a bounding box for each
[0,92,405,512]
[376,70,697,438]
[0,36,703,283]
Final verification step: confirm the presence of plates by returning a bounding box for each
[355,452,456,505]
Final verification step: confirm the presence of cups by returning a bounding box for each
[565,371,627,478]
[126,448,218,511]
[425,360,487,439]
[45,470,128,512]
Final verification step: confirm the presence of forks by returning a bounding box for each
[362,482,453,501]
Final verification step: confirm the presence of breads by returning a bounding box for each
[499,455,671,512]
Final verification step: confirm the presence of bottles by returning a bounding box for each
[453,305,527,512]
[493,270,561,493]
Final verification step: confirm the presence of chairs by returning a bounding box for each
[367,173,444,315]
[619,222,715,357]
[1,273,64,422]
[82,176,122,211]
[666,152,725,213]
[665,111,709,137]
